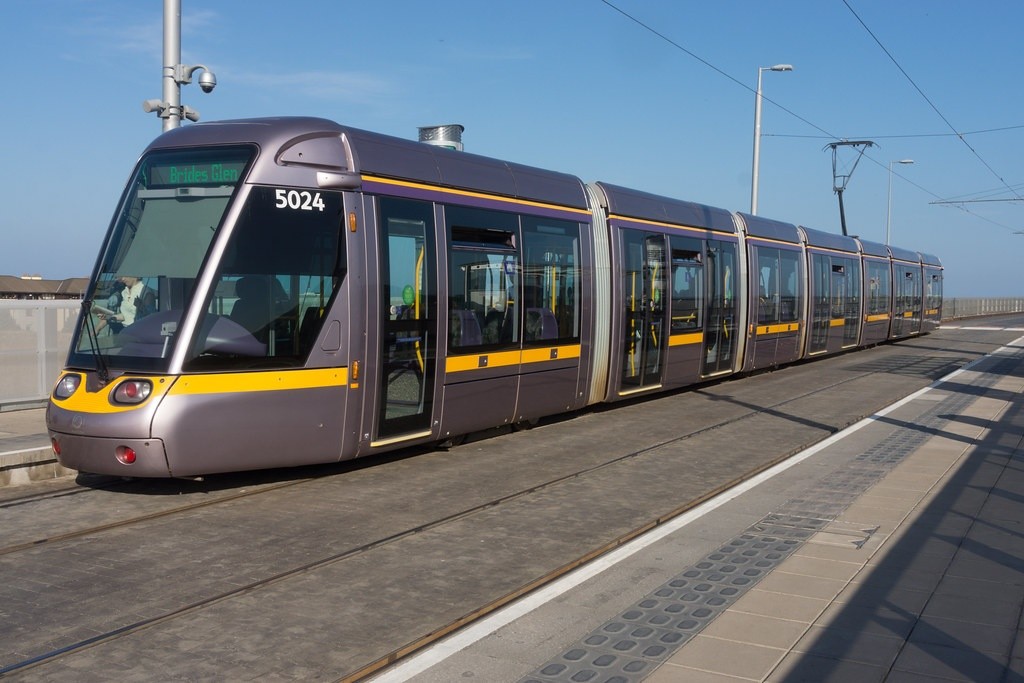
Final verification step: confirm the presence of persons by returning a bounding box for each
[90,273,156,337]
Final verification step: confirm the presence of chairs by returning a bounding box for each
[228,276,269,343]
[528,308,558,340]
[452,310,482,346]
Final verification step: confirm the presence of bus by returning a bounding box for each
[38,112,946,484]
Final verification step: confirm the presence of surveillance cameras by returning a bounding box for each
[198,71,217,93]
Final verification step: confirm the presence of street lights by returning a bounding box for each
[750,62,794,216]
[885,159,915,245]
